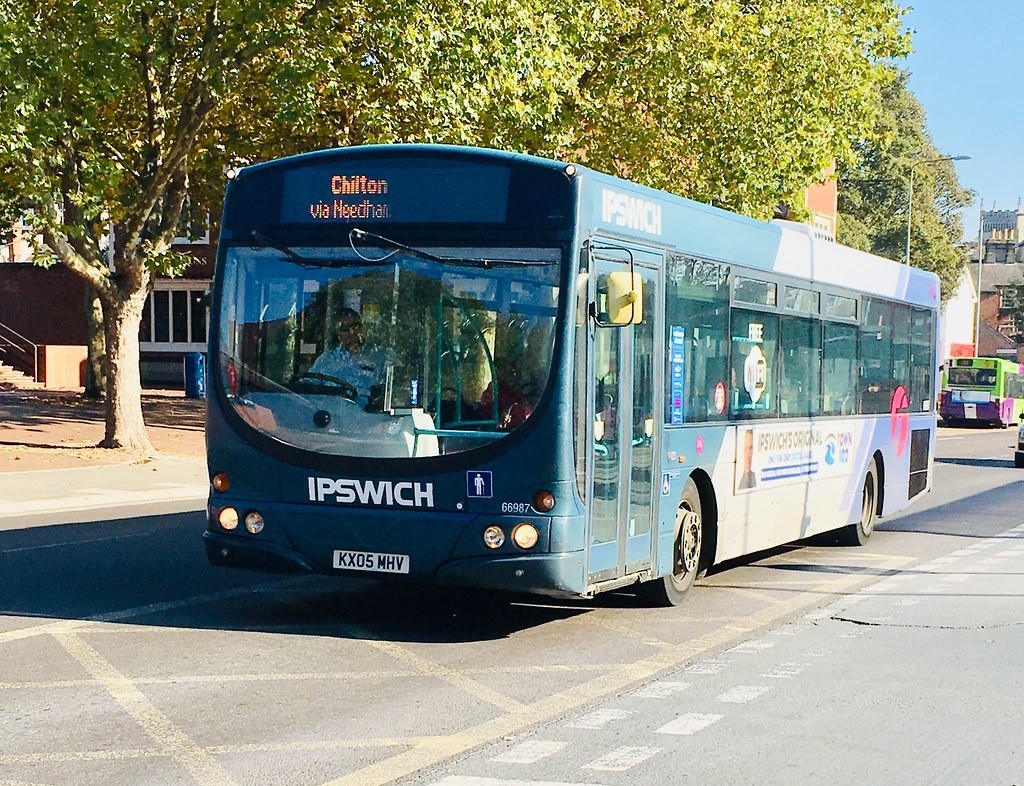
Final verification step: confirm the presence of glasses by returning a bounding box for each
[336,321,363,332]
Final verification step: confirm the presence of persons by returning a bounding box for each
[299,307,396,408]
[480,357,534,428]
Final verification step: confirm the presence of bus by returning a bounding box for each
[937,357,1024,429]
[180,143,941,602]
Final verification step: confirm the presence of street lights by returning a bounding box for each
[906,154,975,264]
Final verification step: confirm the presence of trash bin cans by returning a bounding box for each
[186,352,207,400]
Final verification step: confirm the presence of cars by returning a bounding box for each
[1014,413,1024,468]
